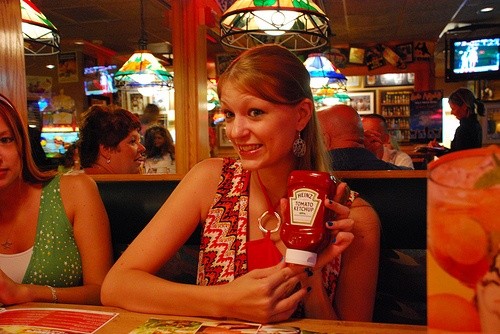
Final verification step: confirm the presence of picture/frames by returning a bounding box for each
[348,90,376,116]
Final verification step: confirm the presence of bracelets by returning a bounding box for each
[44,284,56,302]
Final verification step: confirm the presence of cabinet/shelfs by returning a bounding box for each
[376,86,414,142]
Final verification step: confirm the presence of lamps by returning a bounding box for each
[303,53,353,106]
[220,0,330,52]
[207,77,226,125]
[114,0,174,91]
[19,0,61,56]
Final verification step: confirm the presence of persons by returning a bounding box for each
[0,93,111,305]
[56,89,486,176]
[101,45,382,327]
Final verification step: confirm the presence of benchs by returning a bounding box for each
[89,150,428,326]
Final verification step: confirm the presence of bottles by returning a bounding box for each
[381,105,410,117]
[47,105,52,124]
[73,146,80,171]
[280,170,335,266]
[382,91,411,103]
[412,92,442,105]
[384,118,409,129]
[351,97,370,111]
[131,95,143,111]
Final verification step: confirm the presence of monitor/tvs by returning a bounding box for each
[83,64,118,96]
[445,25,500,83]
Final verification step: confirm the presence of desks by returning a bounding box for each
[0,302,475,334]
